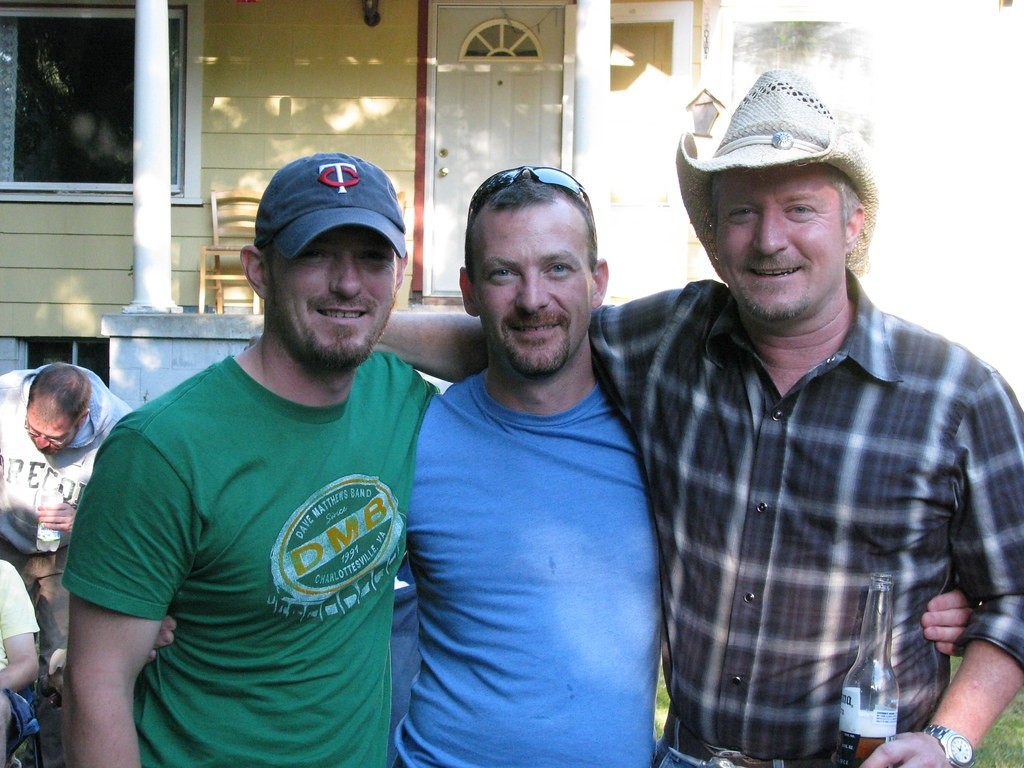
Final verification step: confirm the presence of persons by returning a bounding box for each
[1,363,133,768]
[61,148,441,767]
[371,68,1023,768]
[0,560,41,768]
[146,173,975,768]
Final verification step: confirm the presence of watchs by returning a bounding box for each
[922,723,975,768]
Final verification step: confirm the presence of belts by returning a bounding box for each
[663,713,832,767]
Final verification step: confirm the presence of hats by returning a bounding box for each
[254,152,407,262]
[676,69,878,274]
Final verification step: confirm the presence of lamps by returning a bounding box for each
[362,0,380,26]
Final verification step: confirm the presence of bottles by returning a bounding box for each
[37,477,63,552]
[835,574,900,768]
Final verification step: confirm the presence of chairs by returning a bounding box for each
[198,188,265,314]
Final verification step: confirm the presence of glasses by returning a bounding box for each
[22,415,71,449]
[467,165,593,232]
